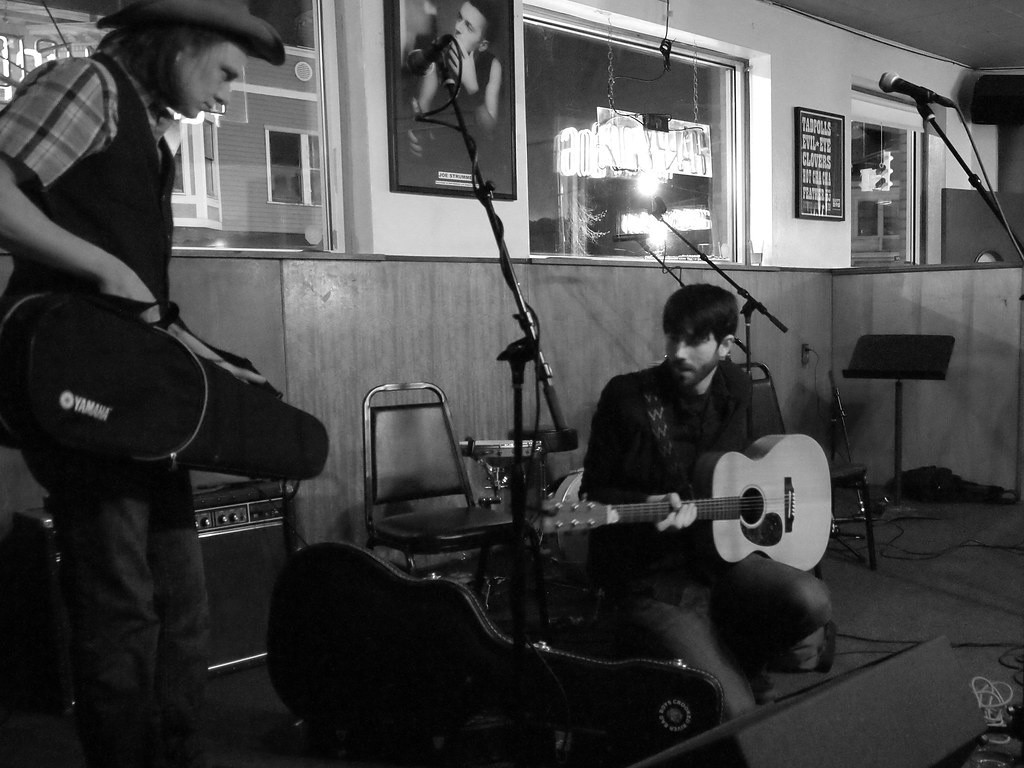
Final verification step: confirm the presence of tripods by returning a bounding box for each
[334,55,618,765]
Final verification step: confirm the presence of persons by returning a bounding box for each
[579,281,834,736]
[0,0,286,768]
[395,1,504,192]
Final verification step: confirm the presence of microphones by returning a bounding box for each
[879,71,956,108]
[406,33,453,77]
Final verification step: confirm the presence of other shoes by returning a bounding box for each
[746,669,776,703]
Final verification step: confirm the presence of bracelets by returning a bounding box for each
[467,89,486,108]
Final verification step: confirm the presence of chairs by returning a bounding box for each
[739,361,877,580]
[362,381,549,628]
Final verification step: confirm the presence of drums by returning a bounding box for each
[458,438,547,461]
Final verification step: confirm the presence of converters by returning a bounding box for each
[642,114,668,132]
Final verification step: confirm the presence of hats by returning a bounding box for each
[96,1,285,66]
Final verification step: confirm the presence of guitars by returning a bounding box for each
[540,433,834,572]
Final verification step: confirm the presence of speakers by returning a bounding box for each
[625,634,988,768]
[187,481,297,679]
[971,74,1024,124]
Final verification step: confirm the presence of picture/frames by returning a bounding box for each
[383,0,518,202]
[794,107,845,222]
